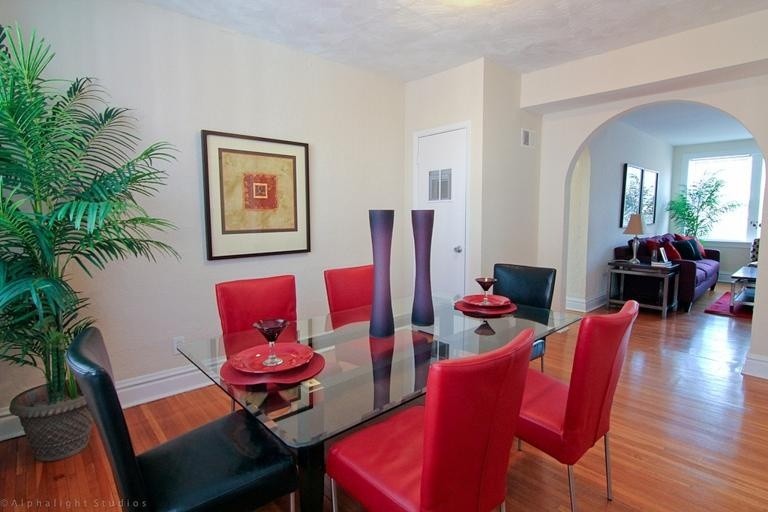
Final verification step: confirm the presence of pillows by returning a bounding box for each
[628,233,705,261]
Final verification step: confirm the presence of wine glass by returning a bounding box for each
[259,390,290,416]
[474,319,495,335]
[475,278,499,306]
[251,318,289,363]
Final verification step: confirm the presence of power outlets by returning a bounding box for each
[171,335,185,355]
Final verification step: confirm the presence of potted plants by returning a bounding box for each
[0,22,182,463]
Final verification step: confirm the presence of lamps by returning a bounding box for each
[622,214,648,265]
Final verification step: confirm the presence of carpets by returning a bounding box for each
[704,291,752,319]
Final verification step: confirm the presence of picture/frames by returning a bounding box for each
[201,130,312,260]
[640,168,659,225]
[620,163,643,228]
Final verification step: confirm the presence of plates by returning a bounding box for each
[219,352,325,386]
[461,293,510,309]
[232,341,314,375]
[455,300,518,315]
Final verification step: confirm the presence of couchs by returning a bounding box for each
[614,246,721,313]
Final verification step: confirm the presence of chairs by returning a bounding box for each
[215,274,299,412]
[63,326,297,511]
[747,238,759,282]
[455,263,557,372]
[324,328,535,512]
[324,263,415,367]
[514,299,640,511]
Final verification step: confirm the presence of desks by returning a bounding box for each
[607,260,680,319]
